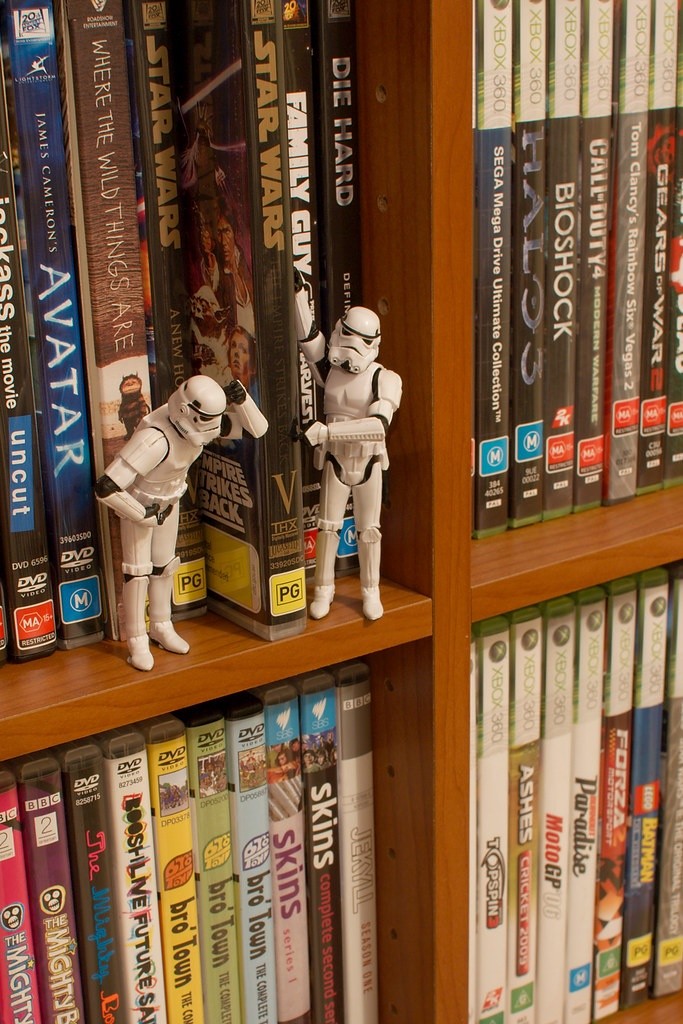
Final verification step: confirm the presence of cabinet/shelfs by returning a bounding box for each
[0,0,683,1024]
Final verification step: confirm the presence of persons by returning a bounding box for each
[294,266,402,619]
[194,196,255,443]
[269,729,338,781]
[94,376,270,671]
